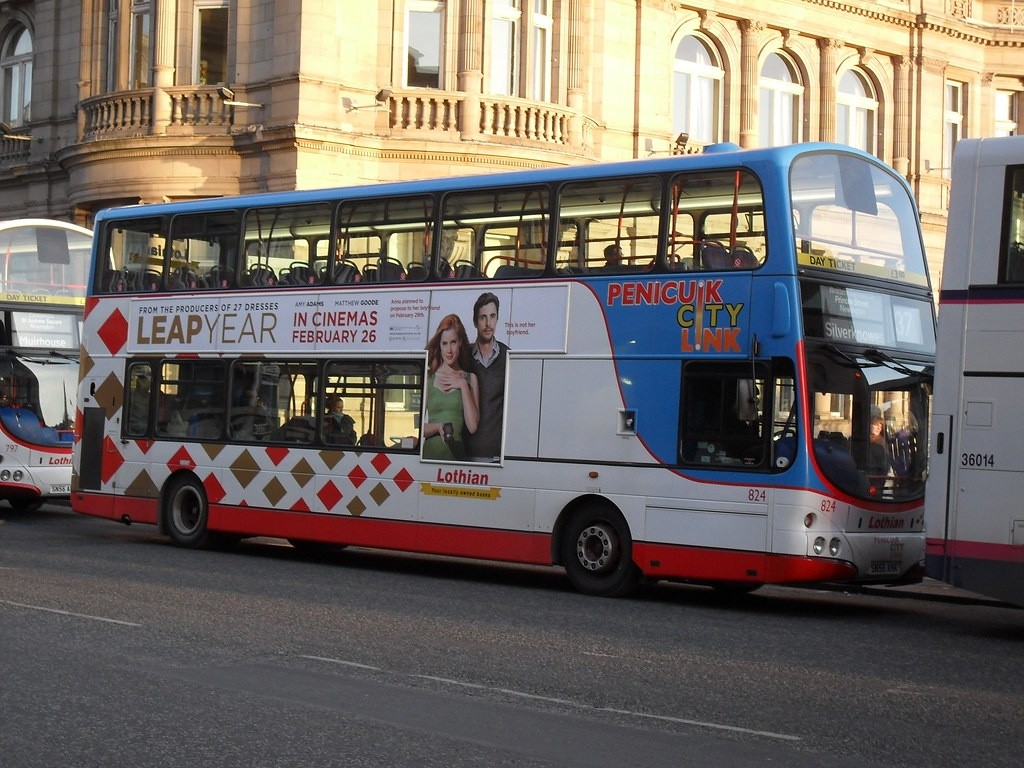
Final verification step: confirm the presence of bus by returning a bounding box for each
[68,138,937,600]
[0,218,116,514]
[918,136,1023,611]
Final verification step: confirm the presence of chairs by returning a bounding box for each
[107,238,760,300]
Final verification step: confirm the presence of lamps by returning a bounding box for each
[648,133,690,156]
[347,88,394,113]
[217,87,261,110]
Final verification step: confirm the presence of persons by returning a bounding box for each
[465,292,511,464]
[322,394,357,446]
[129,375,151,435]
[423,312,479,461]
[870,404,910,498]
[604,245,624,266]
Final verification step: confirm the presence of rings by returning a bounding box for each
[440,433,441,434]
